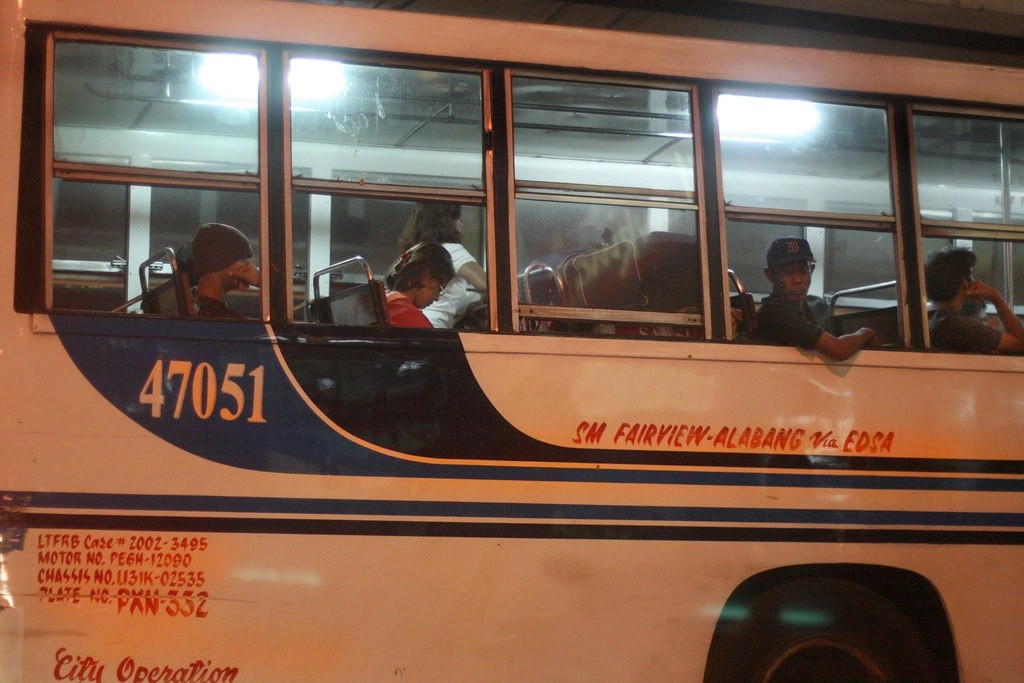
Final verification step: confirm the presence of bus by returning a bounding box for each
[0,1,1024,683]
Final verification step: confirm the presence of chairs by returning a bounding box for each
[303,256,390,327]
[726,269,760,343]
[512,265,579,336]
[138,247,195,319]
[829,281,898,348]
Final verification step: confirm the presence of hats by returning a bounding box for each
[767,236,814,269]
[191,222,254,276]
[176,241,193,270]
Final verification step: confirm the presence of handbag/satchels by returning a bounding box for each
[530,240,650,309]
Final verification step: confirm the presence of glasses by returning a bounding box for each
[439,285,447,296]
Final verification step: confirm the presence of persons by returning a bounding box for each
[384,200,487,328]
[924,246,1024,353]
[174,223,259,318]
[757,236,881,361]
